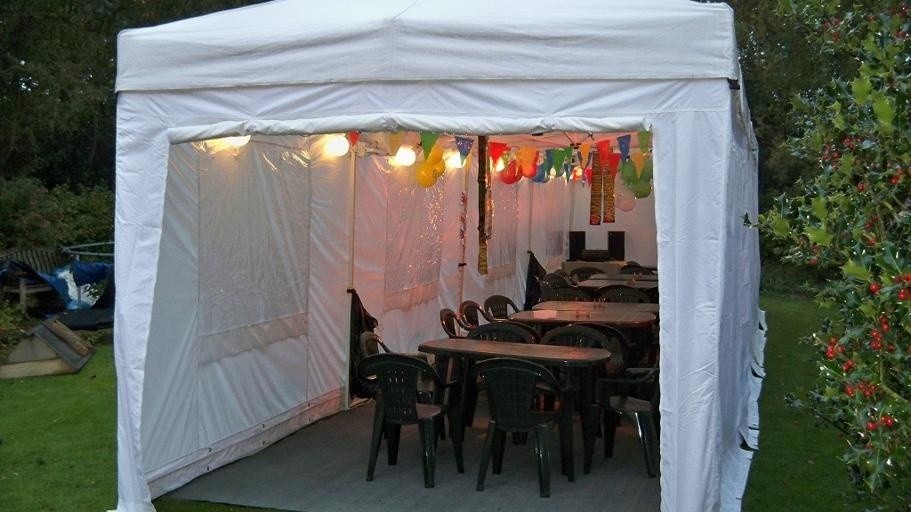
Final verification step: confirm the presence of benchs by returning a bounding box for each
[0,245,68,314]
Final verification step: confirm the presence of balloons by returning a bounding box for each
[500,149,652,211]
[415,144,445,189]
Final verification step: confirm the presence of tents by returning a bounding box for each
[110,1,766,512]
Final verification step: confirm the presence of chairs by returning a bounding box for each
[354,265,660,497]
